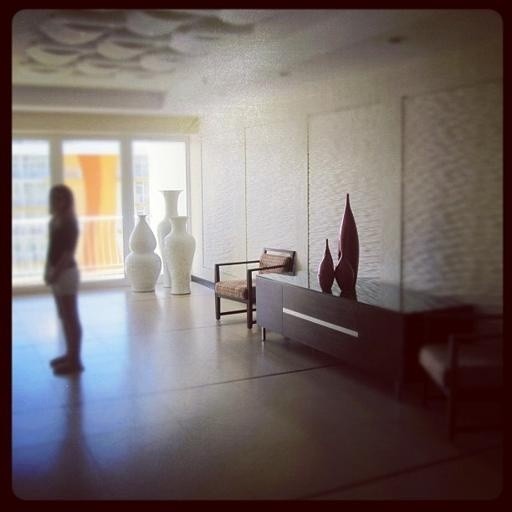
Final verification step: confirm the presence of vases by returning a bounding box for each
[122,189,197,295]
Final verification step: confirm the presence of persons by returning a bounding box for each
[43,186,84,377]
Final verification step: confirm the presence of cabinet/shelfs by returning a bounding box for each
[255,269,478,399]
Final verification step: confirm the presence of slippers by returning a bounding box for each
[50,355,84,374]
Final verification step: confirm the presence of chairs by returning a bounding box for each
[412,309,503,440]
[212,248,297,328]
